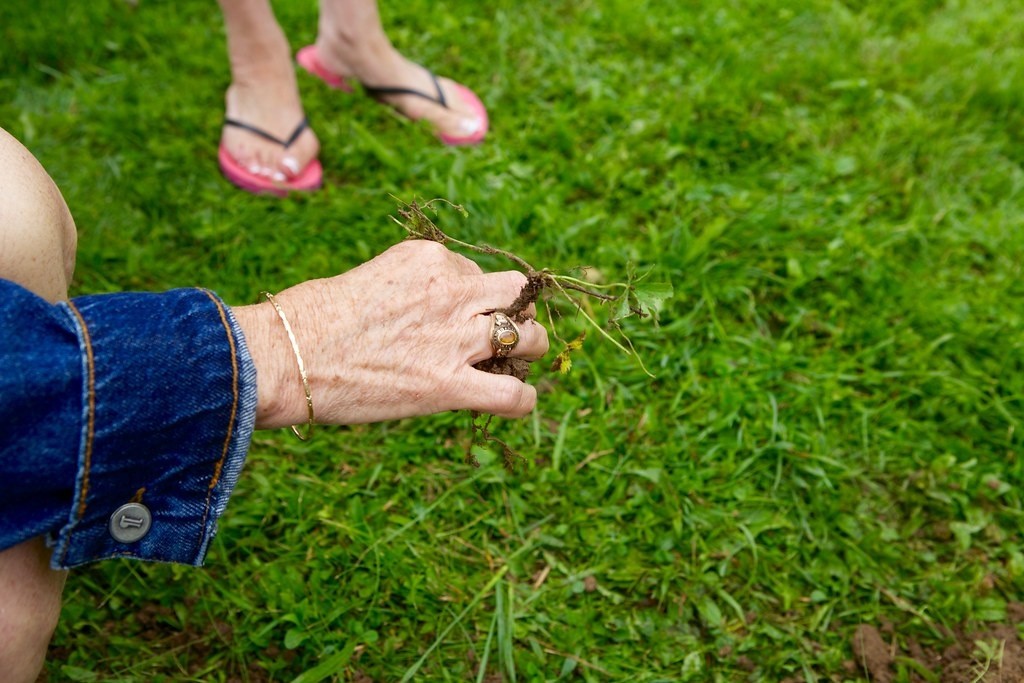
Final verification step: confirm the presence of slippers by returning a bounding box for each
[219,120,323,197]
[295,44,488,146]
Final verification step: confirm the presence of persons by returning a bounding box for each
[216,0,489,198]
[0,134,555,683]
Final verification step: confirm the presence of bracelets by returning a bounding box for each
[262,291,313,441]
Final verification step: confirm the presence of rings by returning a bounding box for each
[490,311,522,362]
